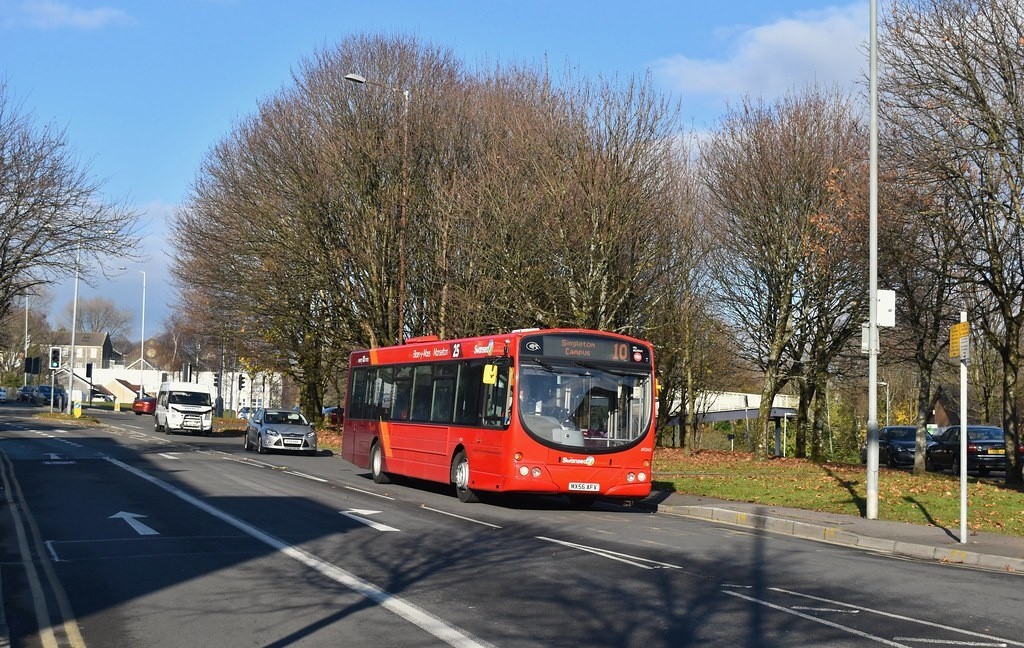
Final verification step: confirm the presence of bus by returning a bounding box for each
[341,324,665,503]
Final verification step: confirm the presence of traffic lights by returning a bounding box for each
[214,373,221,387]
[238,375,246,390]
[50,347,61,369]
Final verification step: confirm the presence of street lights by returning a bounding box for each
[117,265,146,397]
[67,230,119,416]
[1,283,30,386]
[876,382,889,425]
[783,411,798,458]
[345,73,407,346]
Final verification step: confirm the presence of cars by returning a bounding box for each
[33,385,61,404]
[0,387,6,402]
[16,385,35,402]
[131,397,156,416]
[323,407,344,417]
[925,424,1024,477]
[238,407,255,419]
[92,394,112,402]
[859,425,937,470]
[243,407,318,453]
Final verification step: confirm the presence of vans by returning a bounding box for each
[153,382,213,434]
